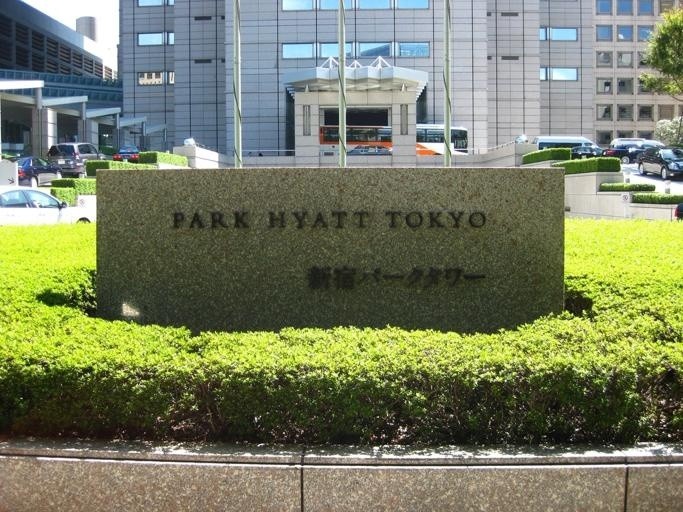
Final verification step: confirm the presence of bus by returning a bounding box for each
[320,124,469,156]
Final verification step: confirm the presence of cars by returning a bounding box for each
[0,142,140,225]
[531,135,683,180]
